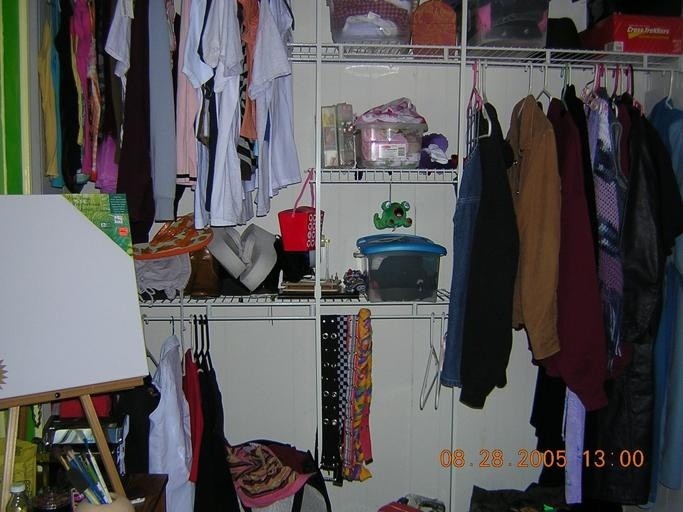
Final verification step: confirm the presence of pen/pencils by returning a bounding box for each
[59,440,112,505]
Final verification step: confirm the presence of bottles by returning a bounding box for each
[5,481,32,512]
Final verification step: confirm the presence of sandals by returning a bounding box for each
[208,224,277,292]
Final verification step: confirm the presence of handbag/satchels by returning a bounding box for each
[278,170,325,253]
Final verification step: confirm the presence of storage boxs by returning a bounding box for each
[456,0,550,63]
[592,14,683,55]
[326,0,413,59]
[48,417,130,492]
[355,233,448,305]
[354,123,426,169]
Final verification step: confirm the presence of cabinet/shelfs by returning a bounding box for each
[20,0,683,512]
[108,473,168,512]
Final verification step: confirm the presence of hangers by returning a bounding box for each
[420,312,439,410]
[465,58,674,144]
[142,314,213,375]
[434,312,448,411]
